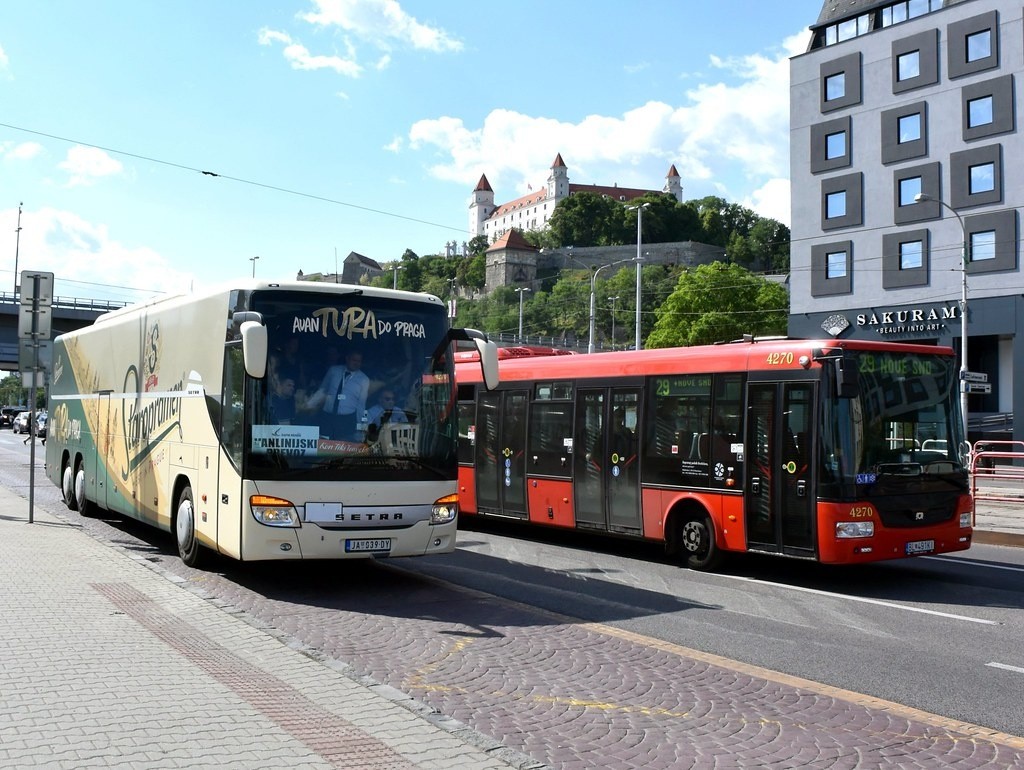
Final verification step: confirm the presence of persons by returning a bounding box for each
[270,336,306,387]
[23,409,41,444]
[591,407,631,490]
[656,397,676,456]
[865,414,913,462]
[262,377,296,425]
[367,389,408,427]
[304,349,370,441]
[41,418,47,445]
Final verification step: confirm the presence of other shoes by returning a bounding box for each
[23,441,26,445]
[41,440,44,445]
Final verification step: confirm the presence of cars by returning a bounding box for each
[0,406,47,438]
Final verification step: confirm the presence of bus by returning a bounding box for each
[430,336,976,574]
[44,279,500,570]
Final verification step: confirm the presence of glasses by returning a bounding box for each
[381,397,397,402]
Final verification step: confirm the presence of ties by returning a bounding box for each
[332,372,352,417]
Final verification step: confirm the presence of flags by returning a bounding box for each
[528,184,532,190]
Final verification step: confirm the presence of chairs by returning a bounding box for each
[673,430,738,485]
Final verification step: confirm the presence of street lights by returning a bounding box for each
[628,202,651,351]
[540,247,650,354]
[250,256,258,278]
[513,286,532,345]
[913,192,967,446]
[606,295,619,350]
[389,264,407,290]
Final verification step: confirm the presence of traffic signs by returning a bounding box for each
[958,371,988,383]
[961,384,993,394]
